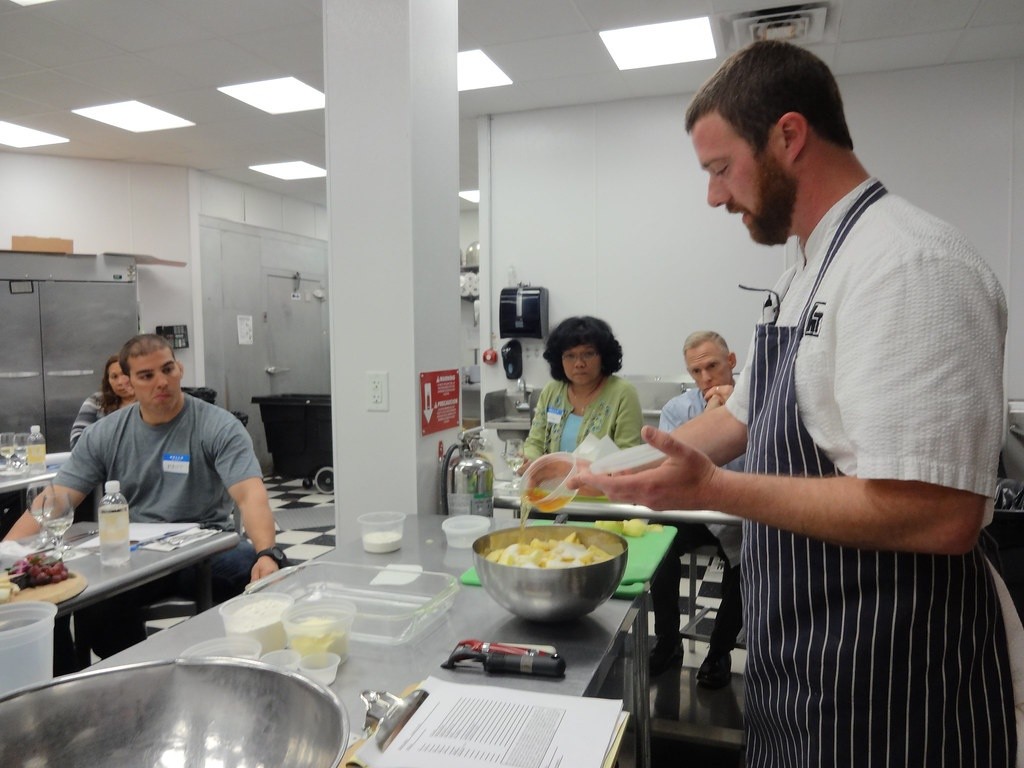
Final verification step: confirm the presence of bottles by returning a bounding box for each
[97,480,131,566]
[27,425,46,475]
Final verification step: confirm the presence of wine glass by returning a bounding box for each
[0,431,29,471]
[503,438,525,488]
[25,479,74,561]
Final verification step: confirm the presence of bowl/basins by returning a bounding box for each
[0,655,351,768]
[299,652,340,686]
[282,593,361,666]
[520,452,581,511]
[356,511,406,553]
[260,649,300,677]
[442,515,490,549]
[472,524,629,623]
[217,592,295,656]
[181,637,260,661]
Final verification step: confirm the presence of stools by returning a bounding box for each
[680,546,747,652]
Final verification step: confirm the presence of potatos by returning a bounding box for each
[485,531,616,568]
[594,518,648,538]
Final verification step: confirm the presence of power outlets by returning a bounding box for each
[367,370,389,412]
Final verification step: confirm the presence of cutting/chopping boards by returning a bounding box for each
[460,520,679,596]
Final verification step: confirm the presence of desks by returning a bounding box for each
[494,480,743,526]
[0,452,106,523]
[73,513,678,768]
[19,524,241,617]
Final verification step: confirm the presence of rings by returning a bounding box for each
[717,387,719,390]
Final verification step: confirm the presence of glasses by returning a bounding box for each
[562,348,600,363]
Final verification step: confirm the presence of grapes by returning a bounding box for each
[11,561,68,590]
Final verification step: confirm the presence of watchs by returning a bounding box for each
[257,545,285,570]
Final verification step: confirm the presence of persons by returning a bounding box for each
[649,331,744,686]
[518,317,644,502]
[527,39,1024,768]
[70,356,136,448]
[3,334,284,659]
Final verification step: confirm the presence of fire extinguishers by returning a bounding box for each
[440,426,494,519]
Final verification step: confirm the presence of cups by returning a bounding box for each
[0,602,58,697]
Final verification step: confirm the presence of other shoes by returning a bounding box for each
[647,642,683,684]
[696,653,733,685]
